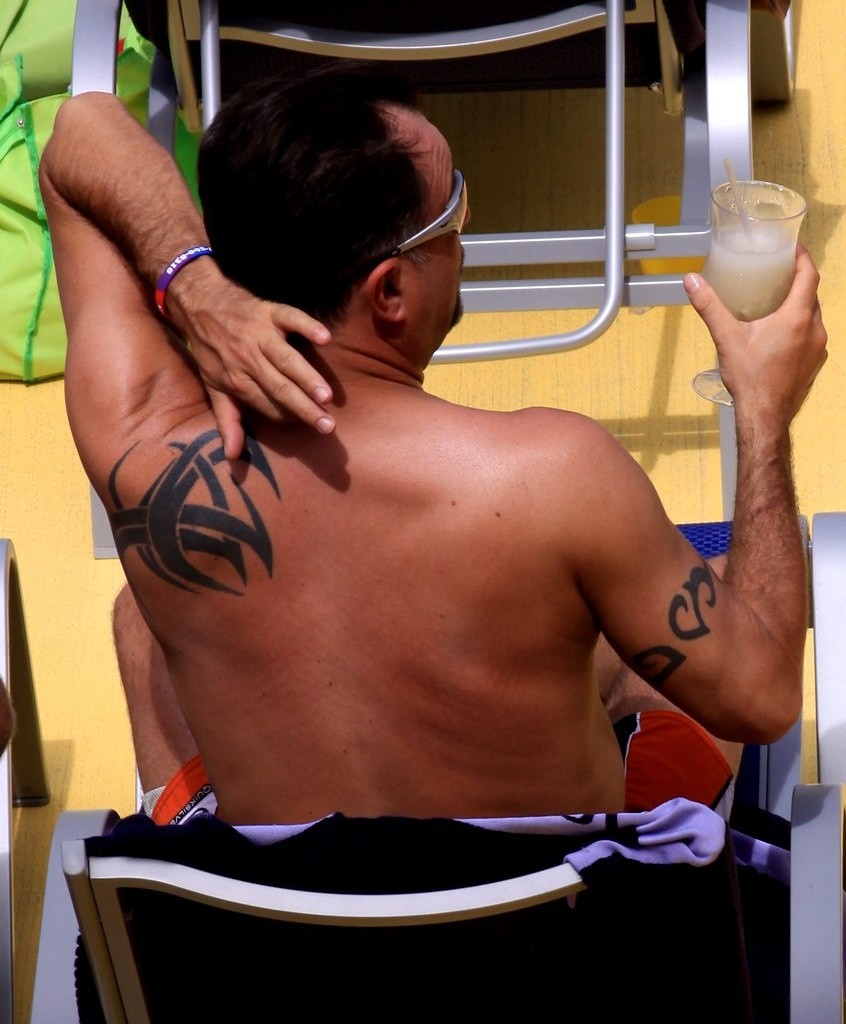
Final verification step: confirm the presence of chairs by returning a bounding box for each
[64,0,795,370]
[27,507,844,1023]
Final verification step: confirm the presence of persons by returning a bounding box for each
[41,68,830,828]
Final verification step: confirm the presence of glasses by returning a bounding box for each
[360,168,467,279]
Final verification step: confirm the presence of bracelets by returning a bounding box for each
[155,246,212,320]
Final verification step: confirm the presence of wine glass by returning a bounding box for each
[687,177,809,410]
